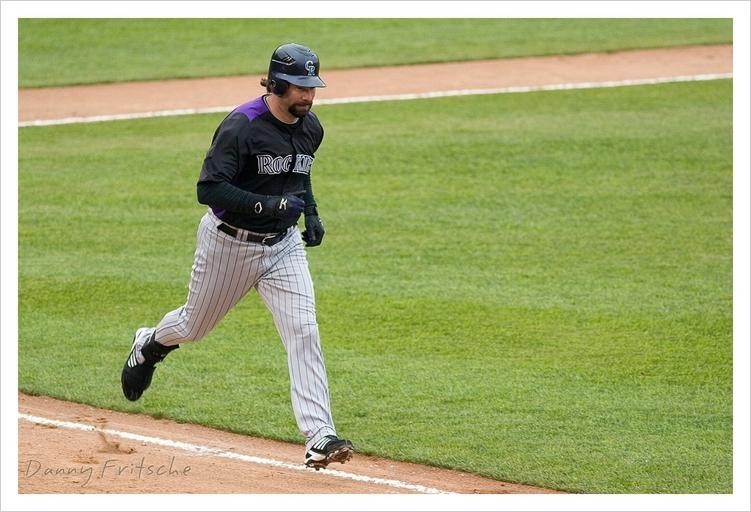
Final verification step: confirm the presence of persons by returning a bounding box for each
[120,41,355,470]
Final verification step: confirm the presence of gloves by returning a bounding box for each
[276,195,305,218]
[301,215,324,246]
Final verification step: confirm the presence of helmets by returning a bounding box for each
[269,44,327,87]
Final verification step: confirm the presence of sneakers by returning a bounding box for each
[304,435,353,470]
[122,327,156,402]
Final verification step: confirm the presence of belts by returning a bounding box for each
[217,222,298,247]
